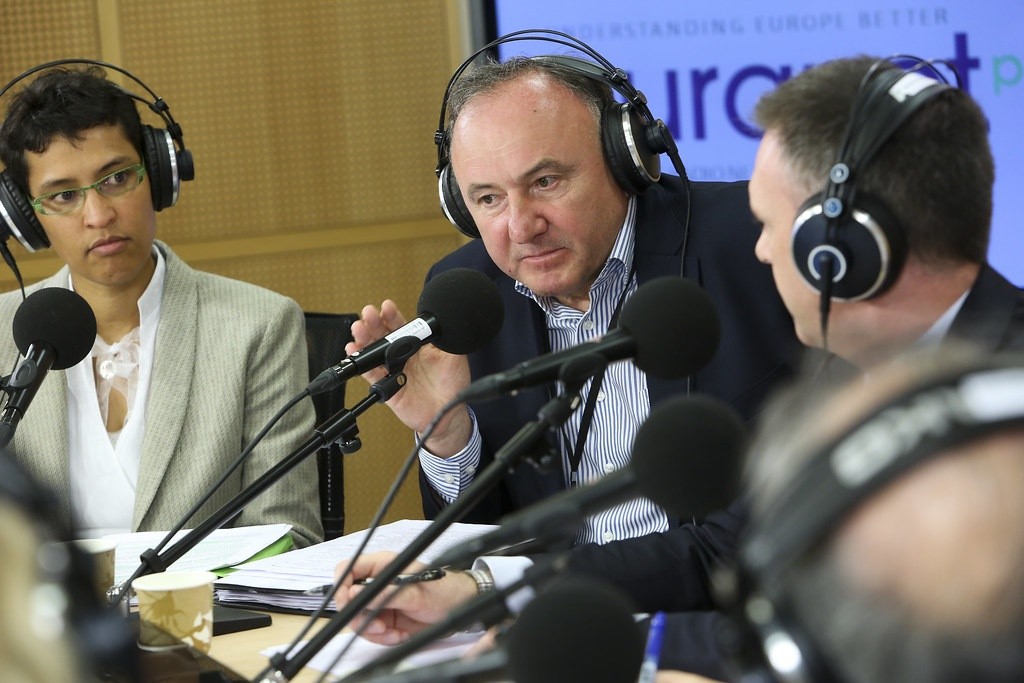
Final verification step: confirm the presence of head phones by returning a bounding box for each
[0,59,192,254]
[1,454,141,683]
[713,359,1024,683]
[433,29,677,239]
[792,54,966,304]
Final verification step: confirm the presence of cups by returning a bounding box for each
[50,539,118,607]
[131,569,218,659]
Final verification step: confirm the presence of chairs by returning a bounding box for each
[303,310,363,544]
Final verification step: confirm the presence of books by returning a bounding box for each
[93,517,502,621]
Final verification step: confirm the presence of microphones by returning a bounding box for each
[0,287,99,450]
[305,268,747,683]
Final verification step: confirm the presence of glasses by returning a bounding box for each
[25,154,146,216]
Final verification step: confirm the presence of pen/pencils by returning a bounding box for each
[637,611,667,683]
[303,568,447,596]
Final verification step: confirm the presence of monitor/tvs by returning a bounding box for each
[465,0,1024,289]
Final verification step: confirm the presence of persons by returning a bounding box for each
[0,59,325,555]
[747,341,1024,683]
[0,447,139,683]
[345,55,1024,683]
[345,58,805,546]
